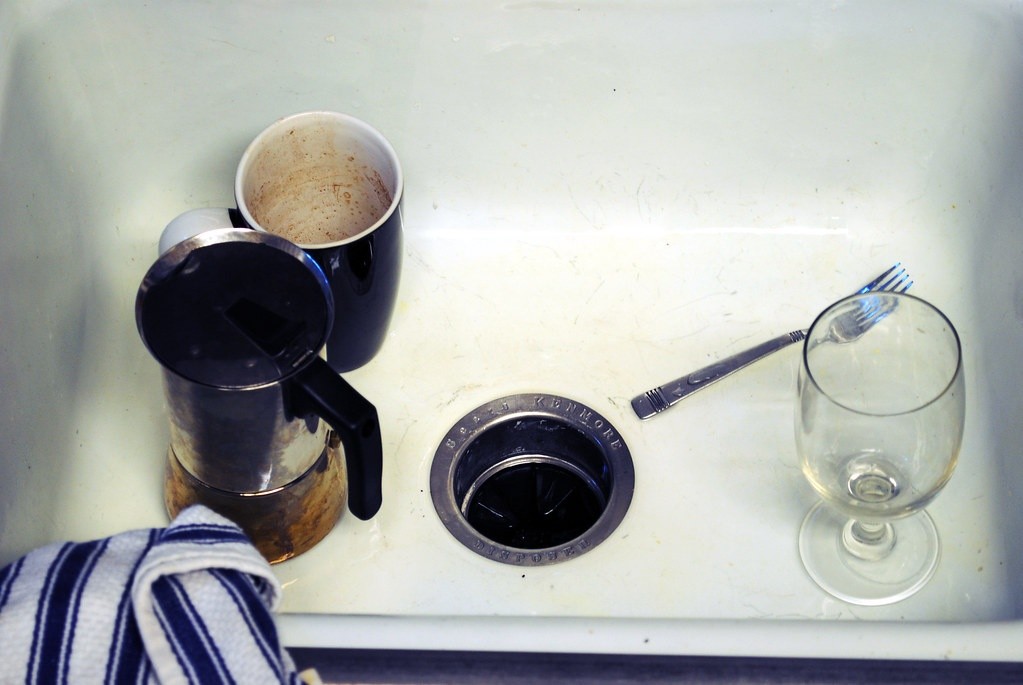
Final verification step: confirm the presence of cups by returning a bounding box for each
[154,112,406,374]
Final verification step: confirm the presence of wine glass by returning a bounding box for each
[792,290,966,604]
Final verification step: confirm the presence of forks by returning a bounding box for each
[631,261,913,420]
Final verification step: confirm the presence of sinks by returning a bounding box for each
[1,1,1023,685]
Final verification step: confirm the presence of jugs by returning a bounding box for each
[134,228,383,562]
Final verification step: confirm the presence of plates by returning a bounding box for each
[158,206,249,258]
